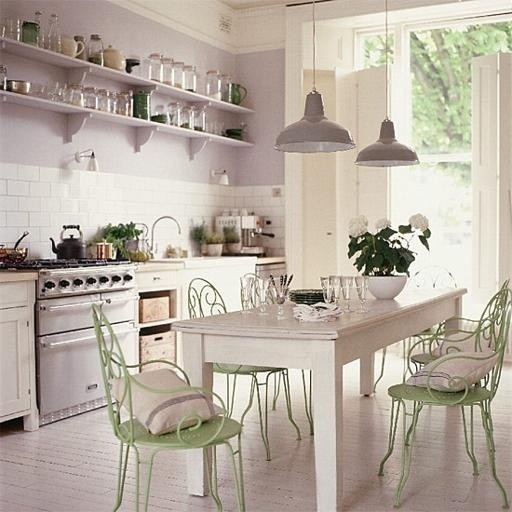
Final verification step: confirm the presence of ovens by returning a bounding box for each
[35,289,140,428]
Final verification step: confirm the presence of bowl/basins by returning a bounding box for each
[7,79,34,96]
[226,128,242,139]
[151,114,168,126]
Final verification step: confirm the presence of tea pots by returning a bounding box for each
[100,44,127,71]
[58,38,84,59]
[228,83,250,105]
[49,225,87,259]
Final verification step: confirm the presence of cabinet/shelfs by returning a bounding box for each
[136,256,257,389]
[0,36,256,160]
[0,269,39,432]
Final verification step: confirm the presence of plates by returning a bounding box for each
[288,289,325,305]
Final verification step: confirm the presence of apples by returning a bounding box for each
[131,251,151,261]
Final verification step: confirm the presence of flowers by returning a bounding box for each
[347,213,431,277]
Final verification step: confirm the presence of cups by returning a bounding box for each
[4,16,40,47]
[96,241,117,260]
[221,208,250,217]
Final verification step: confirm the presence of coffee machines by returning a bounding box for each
[214,215,275,254]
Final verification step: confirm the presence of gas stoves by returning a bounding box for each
[0,258,136,299]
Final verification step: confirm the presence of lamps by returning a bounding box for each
[356,0,419,168]
[75,148,100,171]
[273,0,356,152]
[213,166,233,185]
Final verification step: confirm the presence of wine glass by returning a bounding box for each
[237,276,288,320]
[320,274,369,315]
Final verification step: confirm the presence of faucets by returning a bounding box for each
[149,215,181,260]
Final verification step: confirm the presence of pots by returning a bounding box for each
[0,230,30,265]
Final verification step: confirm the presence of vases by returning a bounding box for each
[366,276,408,300]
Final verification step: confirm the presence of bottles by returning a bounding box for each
[168,100,206,131]
[87,34,105,65]
[0,64,7,91]
[34,8,62,51]
[69,82,150,120]
[147,53,232,104]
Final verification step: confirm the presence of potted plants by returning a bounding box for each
[190,217,242,255]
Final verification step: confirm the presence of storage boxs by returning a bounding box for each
[139,296,168,323]
[140,330,175,372]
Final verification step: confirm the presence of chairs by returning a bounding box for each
[405,278,509,451]
[376,288,512,509]
[91,303,247,512]
[240,273,315,436]
[373,264,456,391]
[187,276,302,461]
